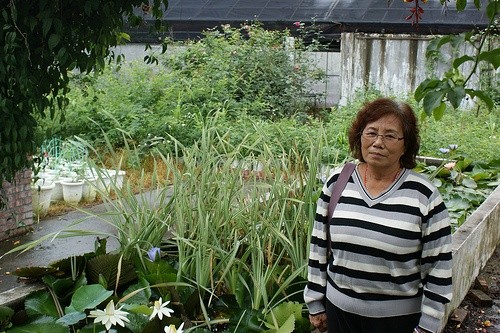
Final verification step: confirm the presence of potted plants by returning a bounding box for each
[33,153,126,217]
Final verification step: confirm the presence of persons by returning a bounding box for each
[303,97,453,332]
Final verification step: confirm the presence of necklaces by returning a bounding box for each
[362,164,401,190]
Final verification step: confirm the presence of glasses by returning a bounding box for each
[360,127,404,143]
